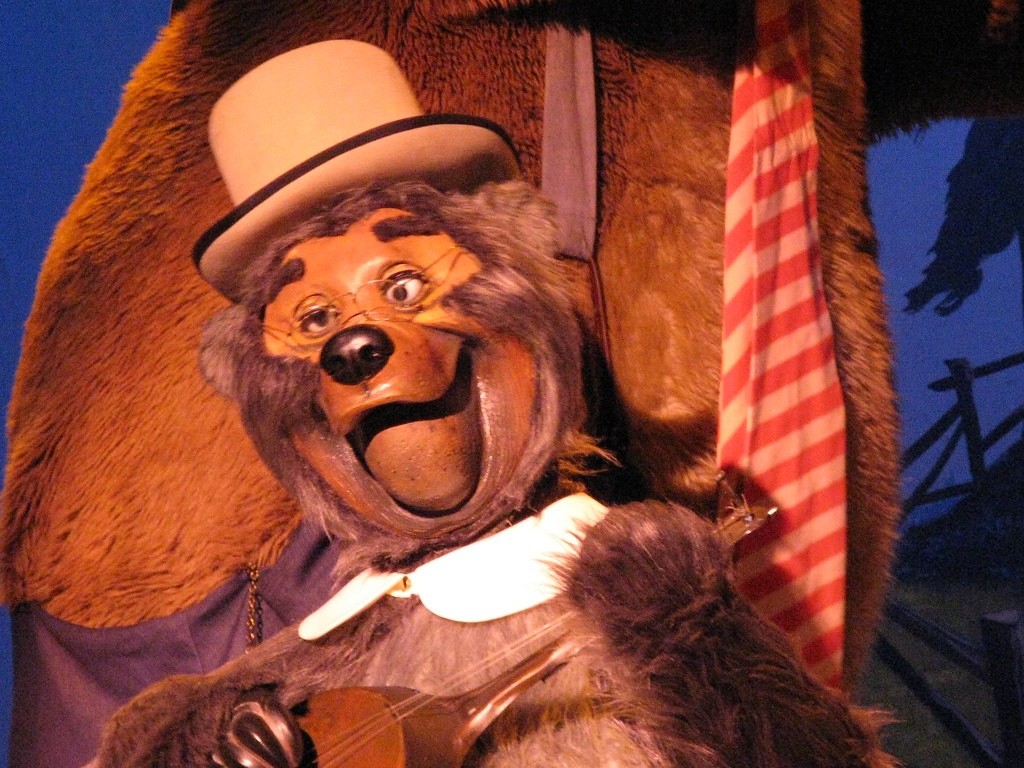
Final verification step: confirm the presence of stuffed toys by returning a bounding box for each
[76,40,909,768]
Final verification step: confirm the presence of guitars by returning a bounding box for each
[285,462,780,768]
